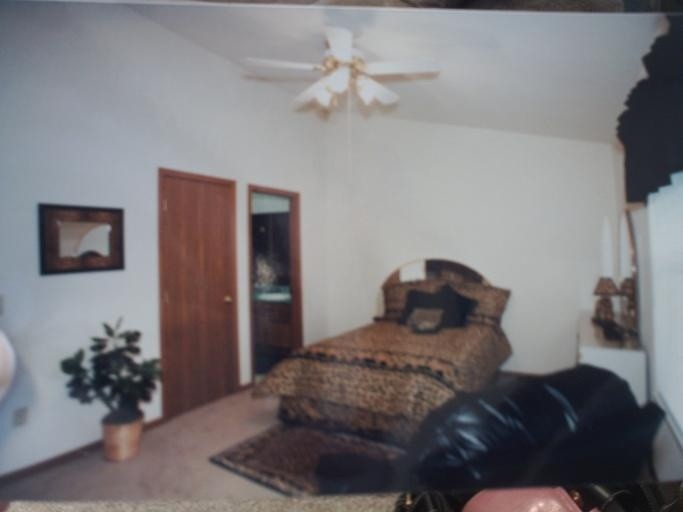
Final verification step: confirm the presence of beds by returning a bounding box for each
[251,258,512,447]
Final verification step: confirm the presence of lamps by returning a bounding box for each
[618,277,637,331]
[592,277,619,324]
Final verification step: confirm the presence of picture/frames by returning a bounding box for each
[38,200,126,276]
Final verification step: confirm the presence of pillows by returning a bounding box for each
[452,282,511,325]
[383,279,451,310]
[397,290,458,327]
[415,321,438,332]
[439,285,477,324]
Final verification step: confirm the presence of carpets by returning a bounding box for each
[208,421,409,497]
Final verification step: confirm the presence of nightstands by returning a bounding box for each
[579,333,649,411]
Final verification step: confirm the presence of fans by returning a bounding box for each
[247,28,440,118]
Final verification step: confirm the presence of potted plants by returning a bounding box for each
[60,316,166,459]
